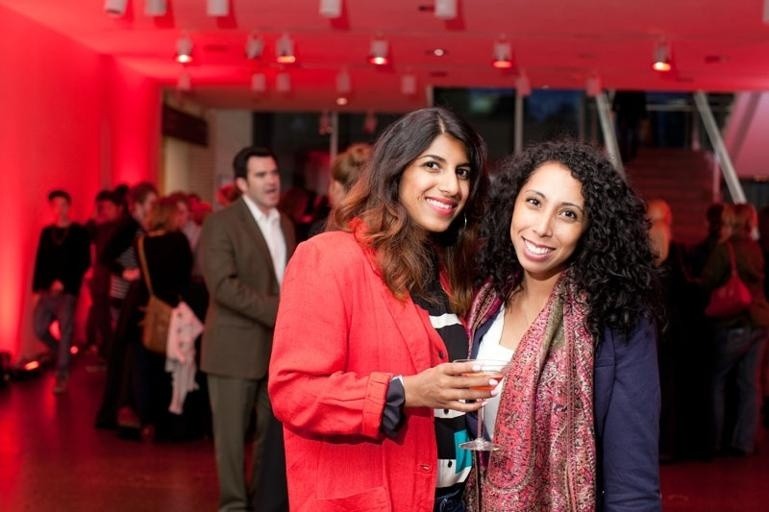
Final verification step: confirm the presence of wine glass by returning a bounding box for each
[451,355,513,458]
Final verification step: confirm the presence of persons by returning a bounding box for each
[327,145,373,207]
[611,91,648,163]
[463,138,661,512]
[200,146,297,511]
[267,106,498,512]
[647,198,766,464]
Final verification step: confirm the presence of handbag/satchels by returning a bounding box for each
[142,296,173,355]
[704,273,753,317]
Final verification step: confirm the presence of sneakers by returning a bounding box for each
[53,378,64,392]
